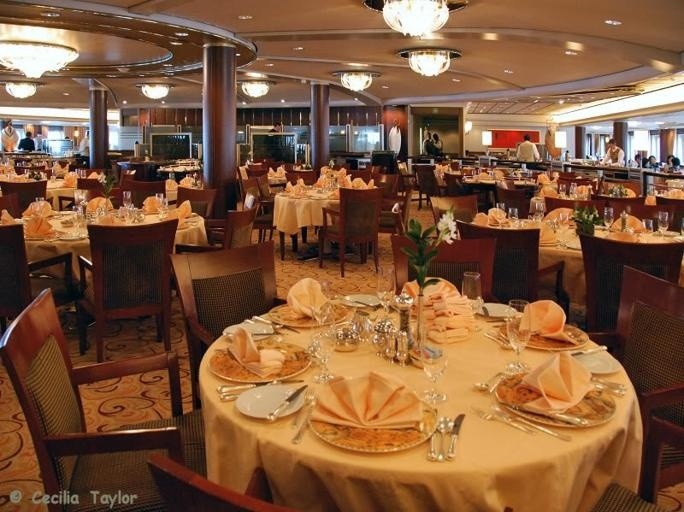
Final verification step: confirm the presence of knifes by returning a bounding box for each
[491,403,573,443]
[562,344,610,356]
[243,316,301,336]
[427,411,467,466]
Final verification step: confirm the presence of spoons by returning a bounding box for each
[469,369,504,393]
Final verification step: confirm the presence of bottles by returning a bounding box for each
[330,312,410,367]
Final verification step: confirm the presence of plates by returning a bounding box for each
[343,292,380,308]
[270,302,351,327]
[484,301,515,319]
[501,320,589,349]
[495,371,616,428]
[309,386,439,453]
[210,339,313,419]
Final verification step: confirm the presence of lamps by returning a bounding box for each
[234,1,469,98]
[0,41,175,100]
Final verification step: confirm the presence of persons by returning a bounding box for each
[422,132,435,156]
[16,131,36,151]
[664,157,681,173]
[263,122,281,155]
[645,155,660,168]
[663,155,680,169]
[596,138,625,168]
[630,153,642,168]
[430,132,442,156]
[514,134,539,162]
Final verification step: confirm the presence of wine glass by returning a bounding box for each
[418,321,450,404]
[506,300,531,371]
[375,265,399,320]
[453,163,669,240]
[1,156,346,240]
[462,270,483,322]
[309,280,337,383]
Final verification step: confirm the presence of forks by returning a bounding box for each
[467,408,534,434]
